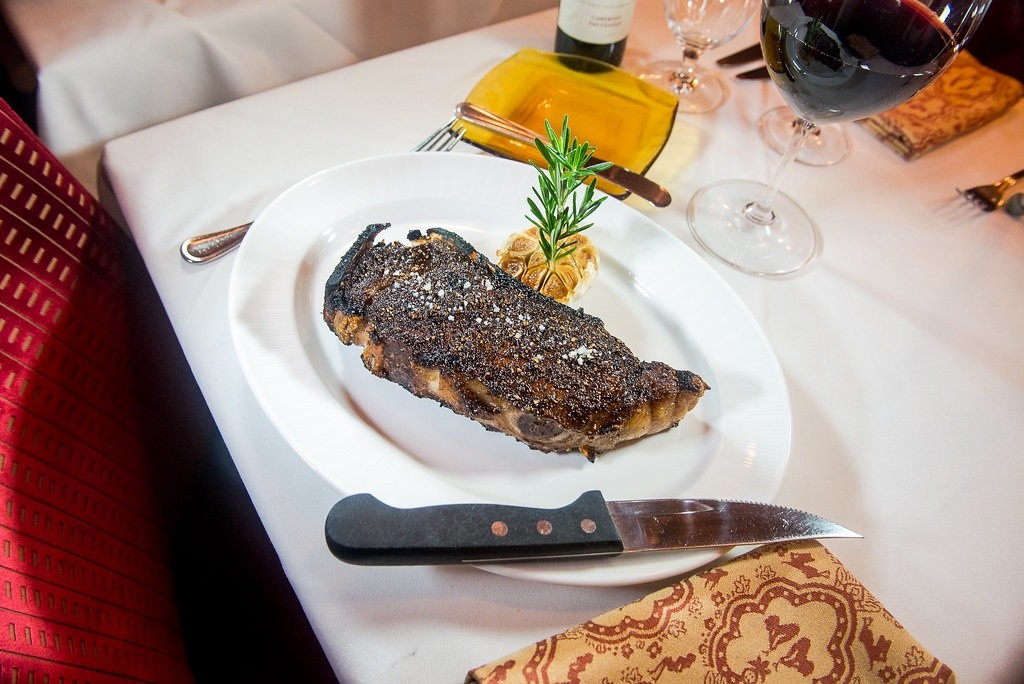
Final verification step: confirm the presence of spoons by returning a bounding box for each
[1006,194,1022,217]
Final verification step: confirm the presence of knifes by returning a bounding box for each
[317,488,866,569]
[454,100,672,208]
[715,41,772,82]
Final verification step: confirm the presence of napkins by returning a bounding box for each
[848,49,1024,161]
[466,542,954,684]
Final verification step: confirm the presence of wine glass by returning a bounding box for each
[642,0,763,116]
[687,1,991,272]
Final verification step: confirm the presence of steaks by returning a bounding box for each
[322,223,713,464]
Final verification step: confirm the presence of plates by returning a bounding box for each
[228,149,793,584]
[447,49,674,198]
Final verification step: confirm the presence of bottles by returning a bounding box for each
[553,0,633,75]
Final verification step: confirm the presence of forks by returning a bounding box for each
[948,167,1024,221]
[178,115,472,264]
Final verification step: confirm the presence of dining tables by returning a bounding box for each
[97,0,1024,684]
